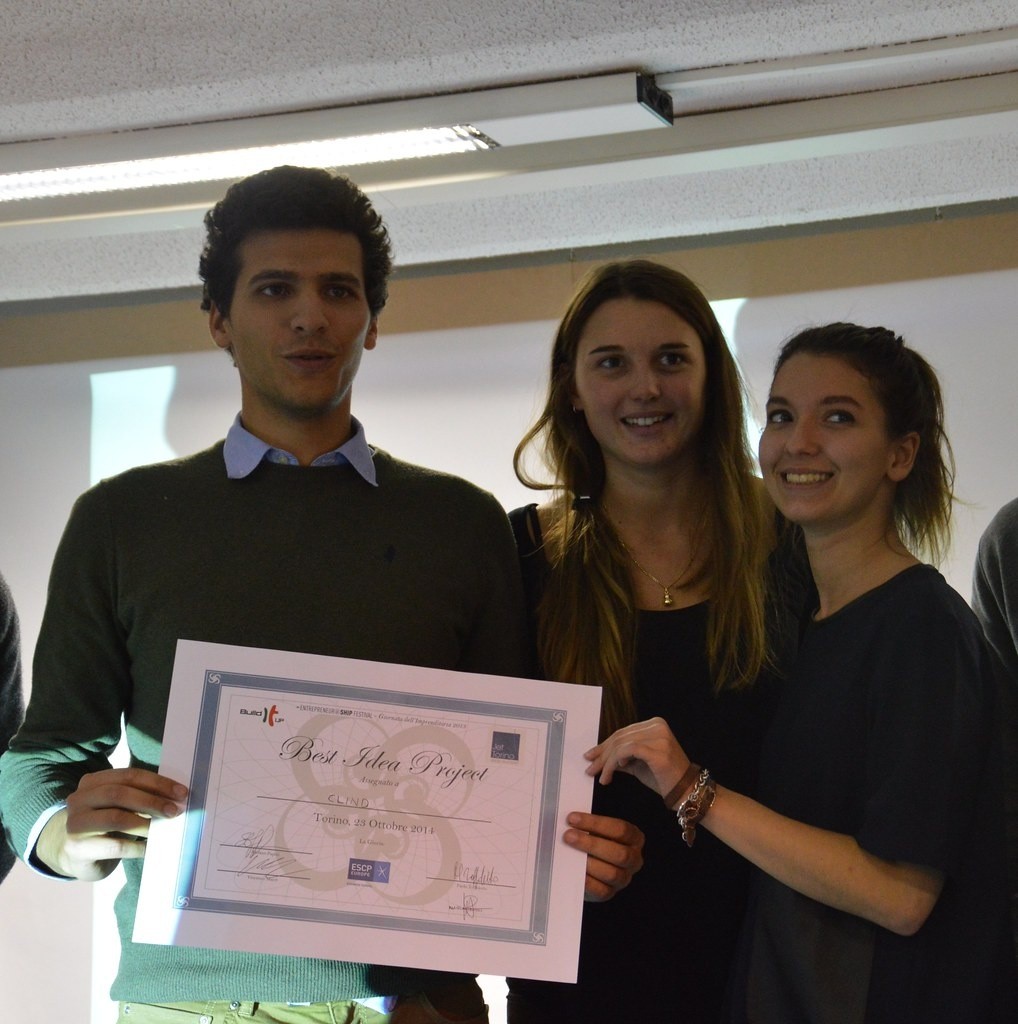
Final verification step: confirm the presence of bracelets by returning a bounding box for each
[663,759,717,851]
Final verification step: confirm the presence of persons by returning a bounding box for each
[577,323,996,1023]
[4,160,645,1024]
[970,497,1018,1023]
[505,252,815,1023]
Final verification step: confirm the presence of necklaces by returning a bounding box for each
[605,480,712,608]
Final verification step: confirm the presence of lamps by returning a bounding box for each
[0,70,677,219]
